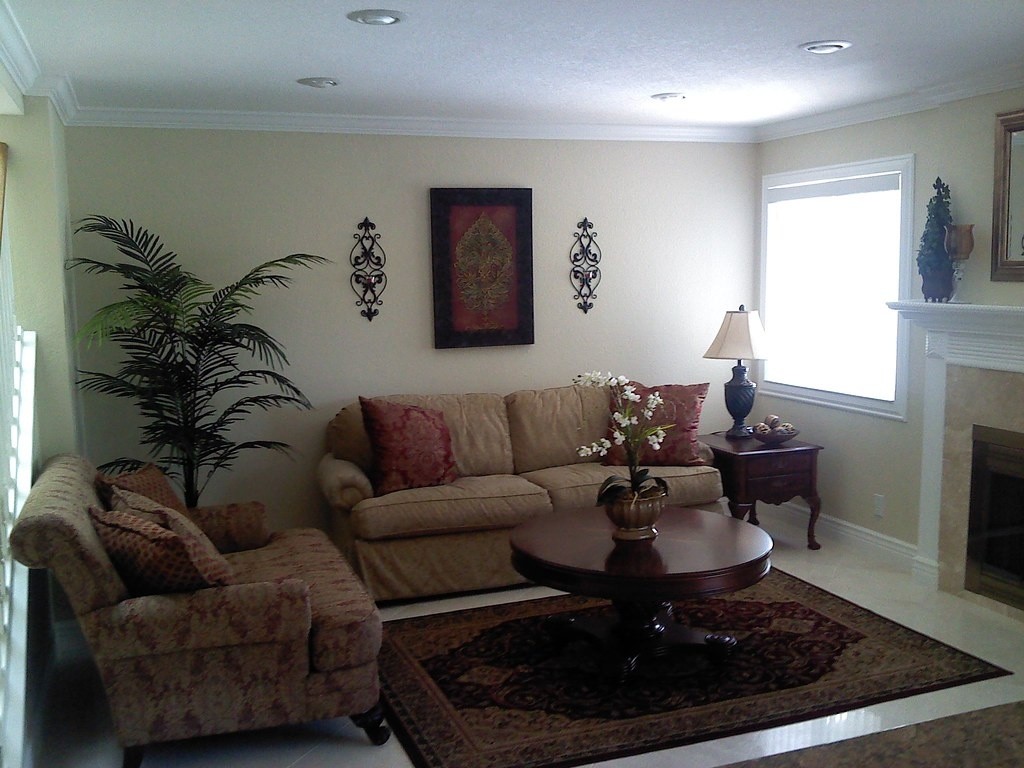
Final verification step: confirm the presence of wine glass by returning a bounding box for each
[942,224,975,303]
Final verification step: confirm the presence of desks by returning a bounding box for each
[507,505,774,677]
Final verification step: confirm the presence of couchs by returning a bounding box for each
[8,453,391,768]
[313,378,732,604]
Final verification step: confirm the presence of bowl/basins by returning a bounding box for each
[752,427,801,445]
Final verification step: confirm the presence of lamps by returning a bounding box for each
[798,39,853,55]
[348,10,407,26]
[702,305,768,439]
[297,77,341,89]
[569,217,603,314]
[350,216,388,322]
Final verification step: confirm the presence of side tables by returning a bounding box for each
[696,433,825,551]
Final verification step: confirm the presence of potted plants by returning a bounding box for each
[917,174,956,303]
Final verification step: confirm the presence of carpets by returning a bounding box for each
[381,567,1017,767]
[721,700,1024,768]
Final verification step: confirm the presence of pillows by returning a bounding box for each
[92,463,191,518]
[598,376,711,466]
[88,504,234,588]
[112,485,235,588]
[358,394,460,496]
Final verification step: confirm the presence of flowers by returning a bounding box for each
[575,385,674,466]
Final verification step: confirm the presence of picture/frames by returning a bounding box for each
[428,187,536,349]
[991,108,1024,283]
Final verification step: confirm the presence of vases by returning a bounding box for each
[603,484,664,539]
